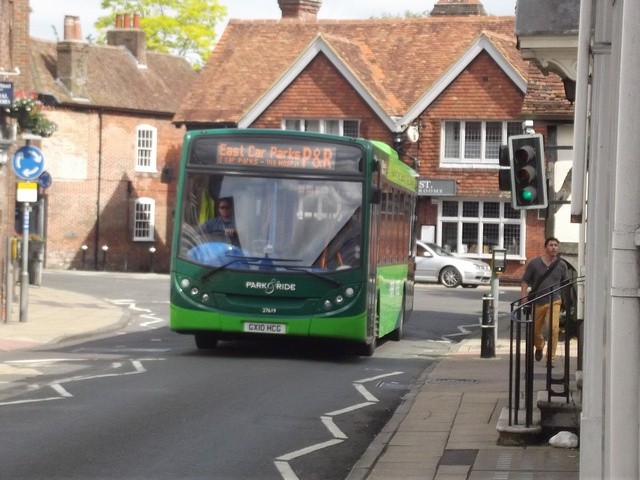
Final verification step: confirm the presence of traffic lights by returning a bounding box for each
[499,170,512,191]
[508,134,548,210]
[499,144,512,191]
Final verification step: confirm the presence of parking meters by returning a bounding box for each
[492,250,506,346]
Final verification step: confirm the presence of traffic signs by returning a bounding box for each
[39,172,52,188]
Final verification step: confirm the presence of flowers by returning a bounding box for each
[3,94,44,130]
[19,114,57,138]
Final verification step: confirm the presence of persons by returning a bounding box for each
[520,237,569,369]
[200,197,238,237]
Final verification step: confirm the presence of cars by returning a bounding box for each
[414,240,492,288]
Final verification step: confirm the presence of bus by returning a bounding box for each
[161,130,420,354]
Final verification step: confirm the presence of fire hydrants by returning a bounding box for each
[480,294,496,356]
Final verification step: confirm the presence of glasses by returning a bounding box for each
[219,204,232,209]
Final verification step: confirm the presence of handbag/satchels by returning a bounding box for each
[523,259,560,314]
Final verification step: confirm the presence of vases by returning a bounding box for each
[0,126,17,140]
[22,128,32,134]
